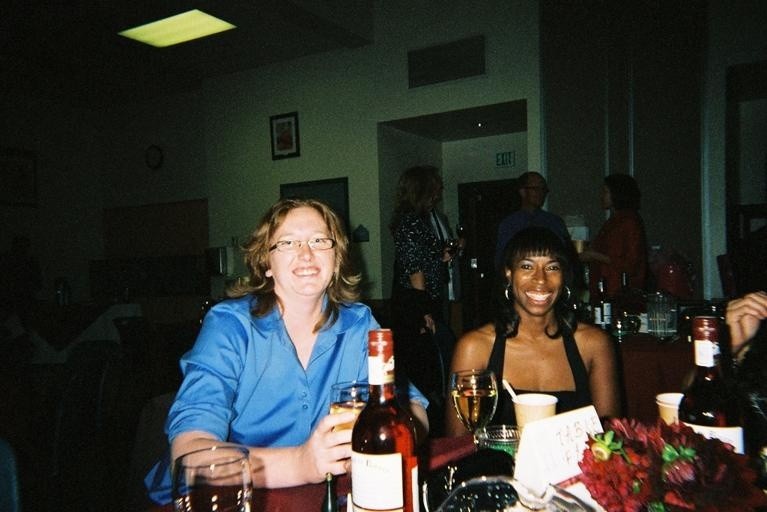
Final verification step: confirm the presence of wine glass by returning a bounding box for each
[444,226,470,262]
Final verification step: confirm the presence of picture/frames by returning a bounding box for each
[267,112,300,161]
[0,145,39,210]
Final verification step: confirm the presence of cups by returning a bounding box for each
[452,370,500,433]
[329,383,368,431]
[655,392,686,425]
[512,392,558,435]
[475,424,523,453]
[170,446,252,511]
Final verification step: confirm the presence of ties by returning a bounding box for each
[430,208,450,283]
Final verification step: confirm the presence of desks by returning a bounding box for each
[152,435,677,510]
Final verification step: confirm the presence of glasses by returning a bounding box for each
[524,186,549,193]
[267,237,335,253]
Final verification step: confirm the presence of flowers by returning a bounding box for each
[578,415,766,511]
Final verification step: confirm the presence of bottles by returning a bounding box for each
[351,327,420,511]
[580,272,720,337]
[320,473,338,511]
[678,317,752,458]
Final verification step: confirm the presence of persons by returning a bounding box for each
[444,225,627,437]
[145,196,430,505]
[678,290,767,456]
